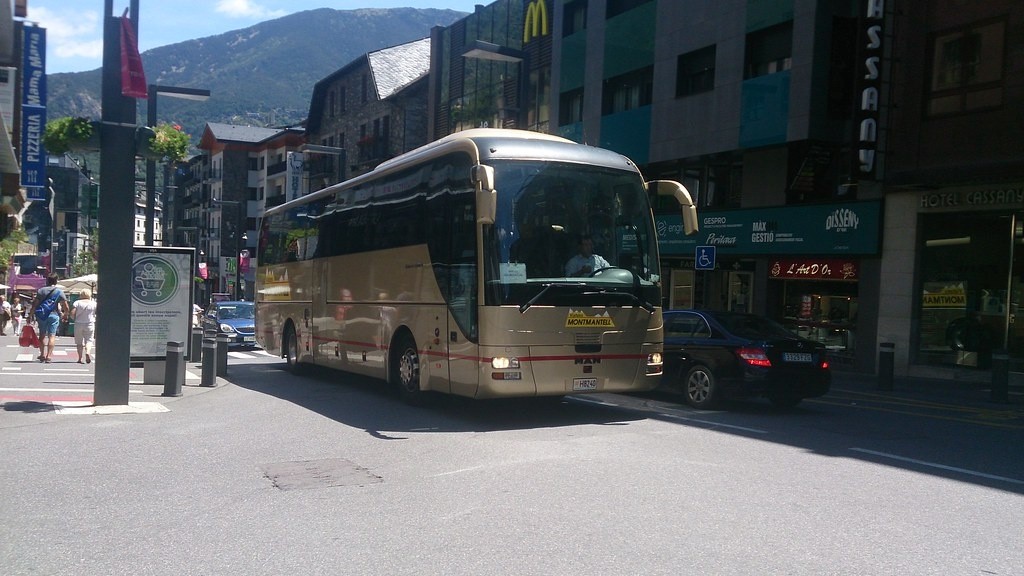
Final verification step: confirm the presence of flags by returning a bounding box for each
[198,263,207,280]
[121,15,147,100]
[238,249,250,273]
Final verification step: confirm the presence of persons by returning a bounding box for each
[373,284,416,348]
[193,304,203,315]
[11,298,25,336]
[564,237,613,279]
[70,289,96,364]
[0,295,11,335]
[27,273,70,363]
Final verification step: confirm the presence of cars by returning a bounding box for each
[201,301,257,349]
[653,311,835,411]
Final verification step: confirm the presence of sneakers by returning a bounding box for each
[39,356,45,363]
[45,355,52,363]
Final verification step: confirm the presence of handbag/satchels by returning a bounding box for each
[19,322,40,349]
[34,287,63,321]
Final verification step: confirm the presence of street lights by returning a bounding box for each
[145,81,211,249]
[174,224,205,305]
[208,197,248,302]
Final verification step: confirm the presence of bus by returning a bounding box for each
[256,128,698,405]
